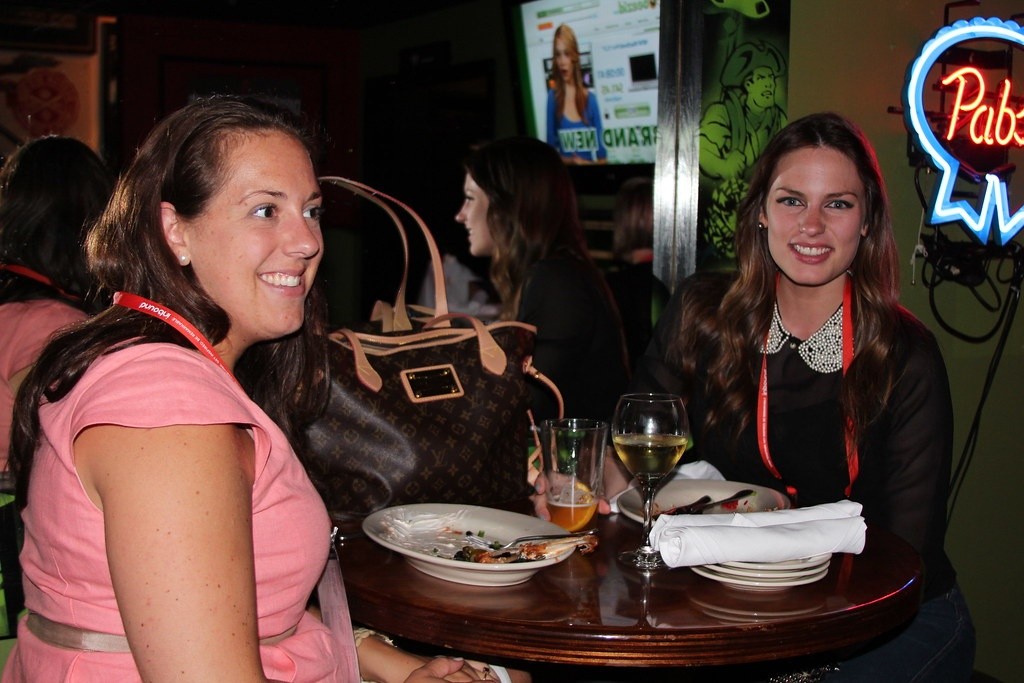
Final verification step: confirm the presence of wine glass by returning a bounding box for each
[611,391,692,573]
[541,418,609,553]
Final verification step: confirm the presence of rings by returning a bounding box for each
[482,667,490,680]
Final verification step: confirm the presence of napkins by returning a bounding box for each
[650,500,867,568]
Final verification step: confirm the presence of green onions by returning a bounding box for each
[434,529,500,562]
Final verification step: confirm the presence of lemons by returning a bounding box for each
[550,481,597,532]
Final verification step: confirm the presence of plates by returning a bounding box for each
[616,477,792,529]
[689,551,831,593]
[362,501,578,588]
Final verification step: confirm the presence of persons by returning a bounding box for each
[0,135,120,498]
[535,111,976,683]
[454,136,628,426]
[0,88,530,683]
[606,189,665,352]
[545,25,608,166]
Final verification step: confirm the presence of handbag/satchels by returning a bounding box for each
[245,175,563,560]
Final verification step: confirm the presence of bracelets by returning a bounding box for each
[354,628,396,683]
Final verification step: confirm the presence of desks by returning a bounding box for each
[325,521,918,667]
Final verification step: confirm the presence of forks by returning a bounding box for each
[671,489,753,515]
[464,528,600,552]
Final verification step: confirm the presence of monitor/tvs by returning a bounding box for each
[513,0,660,183]
